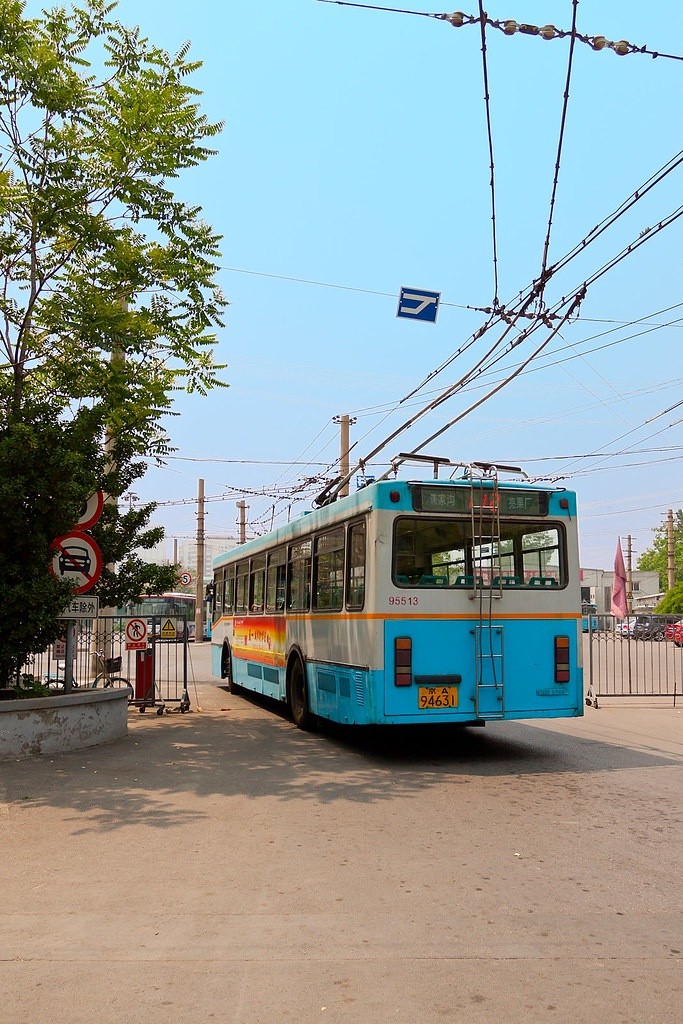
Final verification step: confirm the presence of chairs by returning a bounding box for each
[455,576,483,585]
[529,577,555,586]
[419,575,447,585]
[493,576,520,585]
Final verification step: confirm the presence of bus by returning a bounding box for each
[211,273,587,730]
[582,603,599,632]
[127,592,212,642]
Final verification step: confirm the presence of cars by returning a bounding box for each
[666,619,683,647]
[615,619,638,637]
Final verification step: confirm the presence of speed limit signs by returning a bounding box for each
[180,573,191,585]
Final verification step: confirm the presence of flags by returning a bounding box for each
[611,541,627,619]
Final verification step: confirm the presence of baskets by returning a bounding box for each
[102,656,123,674]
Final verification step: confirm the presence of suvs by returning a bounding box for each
[632,614,681,642]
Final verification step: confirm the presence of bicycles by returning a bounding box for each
[43,648,134,707]
